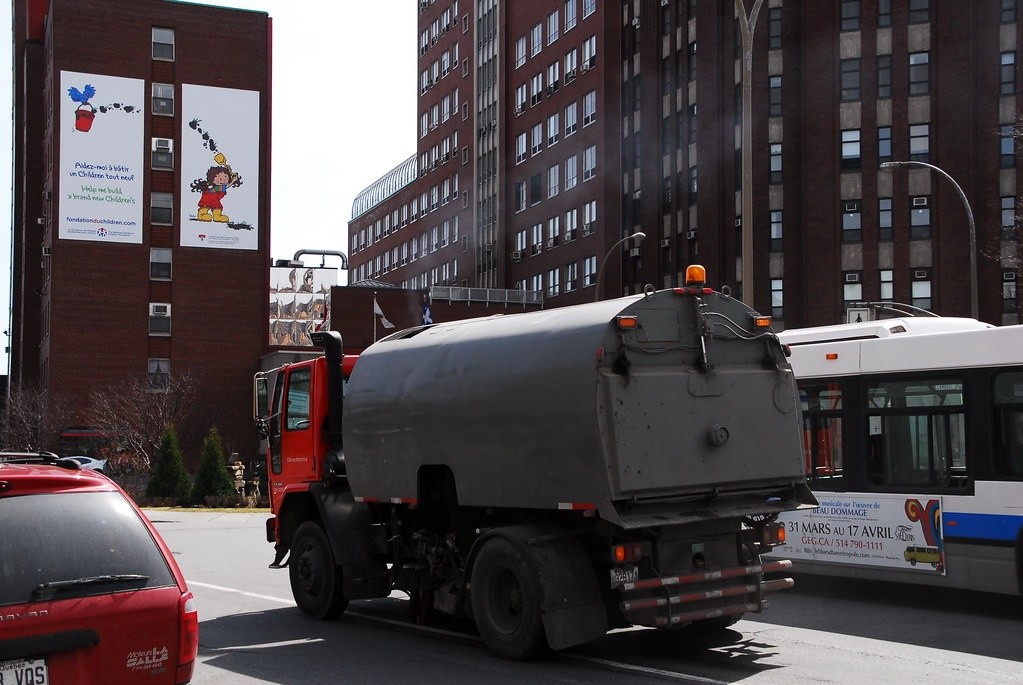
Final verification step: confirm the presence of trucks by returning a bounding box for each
[251,267,819,661]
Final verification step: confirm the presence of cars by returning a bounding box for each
[59,456,104,474]
[0,459,198,685]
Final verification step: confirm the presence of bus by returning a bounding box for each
[756,315,1022,593]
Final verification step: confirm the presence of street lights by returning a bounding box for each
[882,159,979,320]
[595,232,645,302]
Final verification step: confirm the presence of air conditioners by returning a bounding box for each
[421,2,445,42]
[37,218,44,224]
[1004,272,1015,280]
[427,79,434,86]
[42,247,51,256]
[661,239,671,247]
[846,274,859,282]
[41,262,46,268]
[480,64,588,134]
[483,244,493,251]
[452,276,457,281]
[632,18,640,26]
[630,248,640,257]
[913,197,928,206]
[421,123,446,174]
[661,0,669,7]
[846,203,856,211]
[156,139,170,148]
[441,280,446,284]
[153,305,167,315]
[633,192,641,200]
[45,190,51,201]
[687,231,697,240]
[735,219,740,226]
[512,224,590,260]
[915,271,926,278]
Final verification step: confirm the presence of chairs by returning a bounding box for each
[868,433,884,485]
